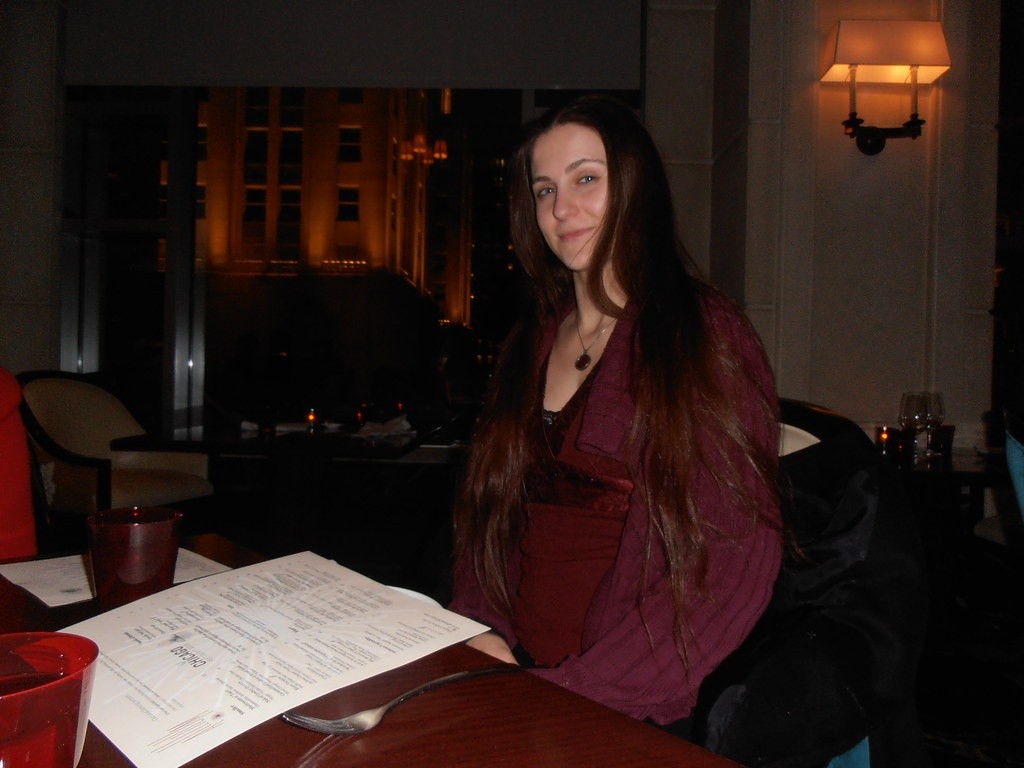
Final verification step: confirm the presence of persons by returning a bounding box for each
[446,92,785,741]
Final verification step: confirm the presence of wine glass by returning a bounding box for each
[899,392,944,458]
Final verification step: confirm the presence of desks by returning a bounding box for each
[843,422,1023,550]
[109,421,473,584]
[0,527,754,768]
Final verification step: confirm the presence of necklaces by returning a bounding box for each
[575,317,616,371]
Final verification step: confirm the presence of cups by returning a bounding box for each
[88,506,184,613]
[0,632,100,768]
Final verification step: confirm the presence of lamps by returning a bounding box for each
[817,20,951,155]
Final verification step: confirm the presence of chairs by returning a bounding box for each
[18,370,228,561]
[700,397,935,767]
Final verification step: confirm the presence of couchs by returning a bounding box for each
[199,268,476,436]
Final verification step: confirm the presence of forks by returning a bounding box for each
[282,663,523,735]
